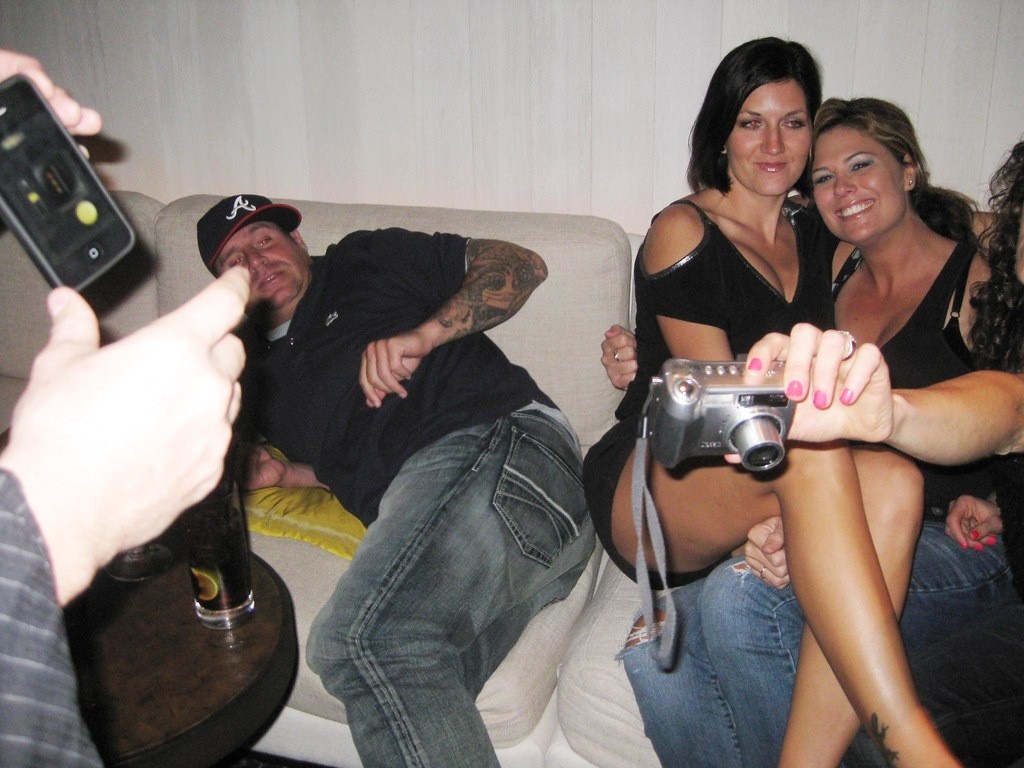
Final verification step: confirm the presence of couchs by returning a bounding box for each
[0,194,663,768]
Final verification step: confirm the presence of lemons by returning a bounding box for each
[190,567,218,601]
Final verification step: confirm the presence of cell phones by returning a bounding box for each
[0,74,136,293]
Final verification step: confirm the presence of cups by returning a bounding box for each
[184,479,256,630]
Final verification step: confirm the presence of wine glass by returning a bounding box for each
[107,547,174,583]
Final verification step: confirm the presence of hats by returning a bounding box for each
[198,194,301,270]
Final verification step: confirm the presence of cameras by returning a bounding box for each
[647,358,796,471]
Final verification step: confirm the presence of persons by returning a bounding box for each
[582,38,996,768]
[196,194,597,768]
[601,101,990,768]
[0,46,250,768]
[726,139,1024,768]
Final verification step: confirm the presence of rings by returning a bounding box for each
[760,566,766,579]
[614,348,619,361]
[839,331,856,360]
